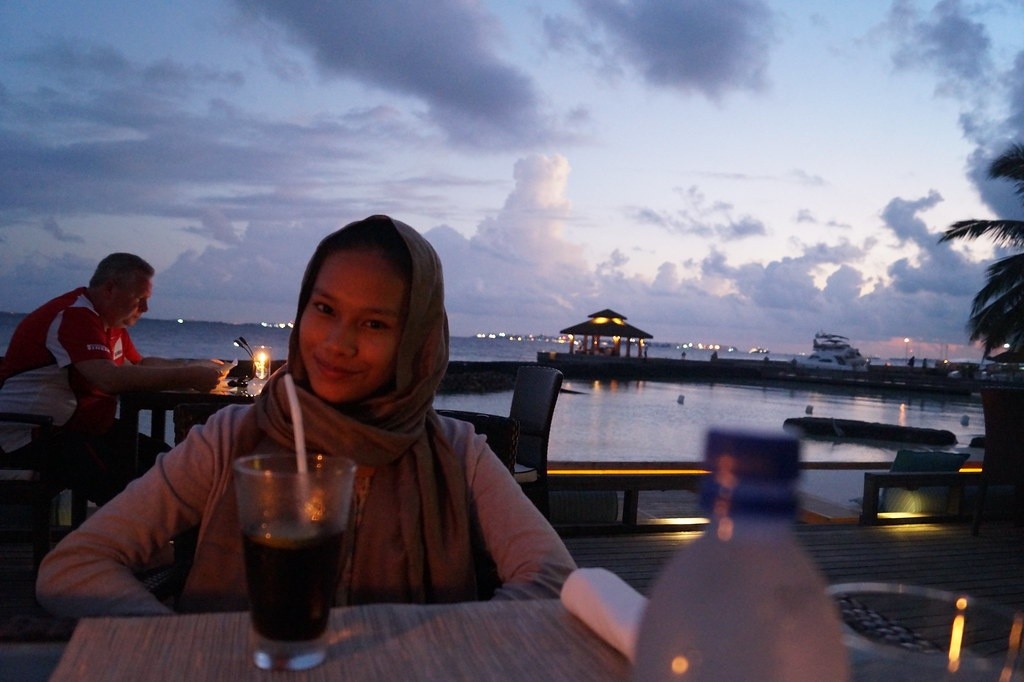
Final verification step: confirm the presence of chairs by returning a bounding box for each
[1,408,92,586]
[431,363,563,532]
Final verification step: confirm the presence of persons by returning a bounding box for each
[36,214,579,618]
[0,252,174,507]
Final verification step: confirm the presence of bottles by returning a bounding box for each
[634,425,849,682]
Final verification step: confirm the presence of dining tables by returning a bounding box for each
[51,591,662,680]
[121,388,272,473]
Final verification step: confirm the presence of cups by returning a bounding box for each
[232,452,360,670]
[252,346,272,385]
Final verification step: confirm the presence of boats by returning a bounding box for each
[799,333,877,376]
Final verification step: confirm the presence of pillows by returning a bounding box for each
[880,448,969,523]
